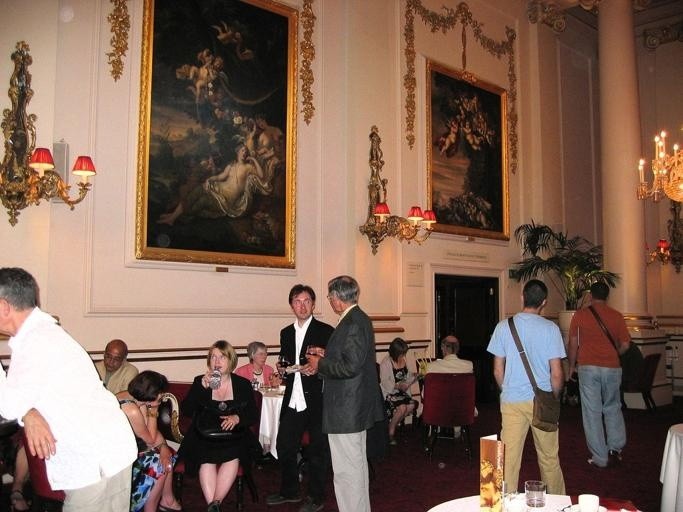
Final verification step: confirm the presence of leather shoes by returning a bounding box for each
[608,450,622,464]
[585,457,599,467]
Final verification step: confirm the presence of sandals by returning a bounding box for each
[158,504,187,512]
[10,490,30,512]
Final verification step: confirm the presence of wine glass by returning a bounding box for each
[275,356,288,380]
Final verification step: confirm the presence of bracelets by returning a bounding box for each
[145,411,159,418]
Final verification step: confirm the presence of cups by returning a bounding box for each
[525,481,546,507]
[207,368,221,388]
[579,494,600,512]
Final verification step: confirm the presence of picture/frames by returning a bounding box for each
[426,58,511,242]
[135,0,298,268]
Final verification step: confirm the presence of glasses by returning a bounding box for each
[293,300,312,307]
[327,295,333,302]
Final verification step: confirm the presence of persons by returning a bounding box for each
[427,335,479,437]
[378,337,415,445]
[0,266,138,512]
[567,281,630,469]
[264,284,335,512]
[157,25,282,235]
[234,341,274,389]
[8,441,30,512]
[485,278,566,497]
[305,275,376,512]
[180,340,259,512]
[94,340,139,395]
[114,370,182,512]
[439,92,498,160]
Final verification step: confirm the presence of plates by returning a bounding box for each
[571,504,607,512]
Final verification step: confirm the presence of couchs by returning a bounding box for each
[158,381,194,444]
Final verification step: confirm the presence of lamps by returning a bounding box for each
[636,127,683,202]
[359,124,436,256]
[0,41,96,227]
[646,199,683,274]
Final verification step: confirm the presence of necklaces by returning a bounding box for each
[215,382,228,412]
[252,368,264,376]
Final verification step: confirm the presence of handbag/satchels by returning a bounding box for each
[507,316,558,432]
[200,426,233,441]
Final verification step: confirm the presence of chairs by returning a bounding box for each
[376,362,419,448]
[620,353,662,413]
[173,390,262,512]
[421,373,476,457]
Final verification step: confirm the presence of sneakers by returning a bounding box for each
[299,496,324,512]
[389,435,396,445]
[265,494,302,505]
[208,502,220,512]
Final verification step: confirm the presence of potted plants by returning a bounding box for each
[514,217,624,350]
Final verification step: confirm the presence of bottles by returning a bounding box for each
[208,367,224,389]
